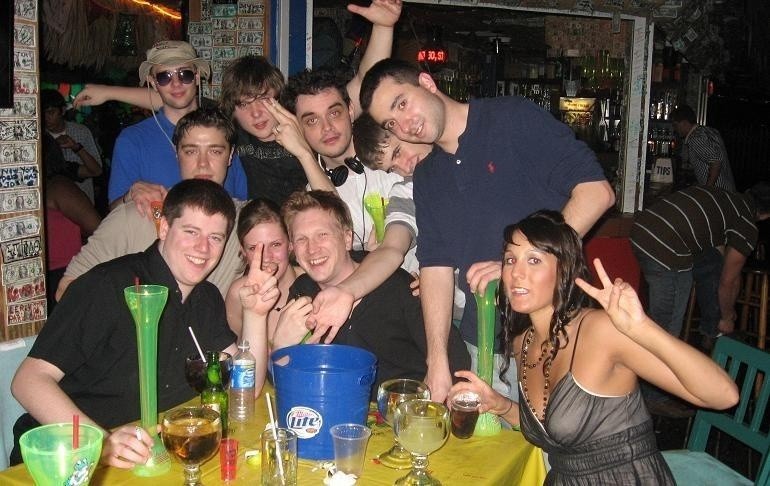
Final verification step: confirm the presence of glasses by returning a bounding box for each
[152,70,197,86]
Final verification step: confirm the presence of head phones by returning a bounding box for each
[318,153,364,187]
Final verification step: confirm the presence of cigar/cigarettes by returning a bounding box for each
[714,332,723,339]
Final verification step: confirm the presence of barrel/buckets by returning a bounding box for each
[268,343,379,461]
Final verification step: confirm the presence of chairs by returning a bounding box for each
[657,329,768,483]
[2,337,46,468]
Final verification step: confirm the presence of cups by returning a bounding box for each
[361,187,388,244]
[260,427,299,486]
[329,423,372,479]
[655,102,669,121]
[448,391,483,440]
[220,436,239,482]
[124,282,174,477]
[463,278,504,437]
[18,421,104,486]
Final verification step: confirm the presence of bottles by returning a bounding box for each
[583,49,627,90]
[610,129,679,155]
[201,351,229,437]
[228,339,256,426]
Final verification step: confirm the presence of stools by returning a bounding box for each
[683,278,704,347]
[730,254,769,396]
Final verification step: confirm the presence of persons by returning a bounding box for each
[668,105,736,195]
[626,181,770,420]
[447,210,741,486]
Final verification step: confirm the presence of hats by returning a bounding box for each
[137,39,212,87]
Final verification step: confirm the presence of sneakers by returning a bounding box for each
[647,398,694,418]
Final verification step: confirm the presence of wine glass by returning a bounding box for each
[159,407,223,485]
[392,398,451,485]
[375,378,431,470]
